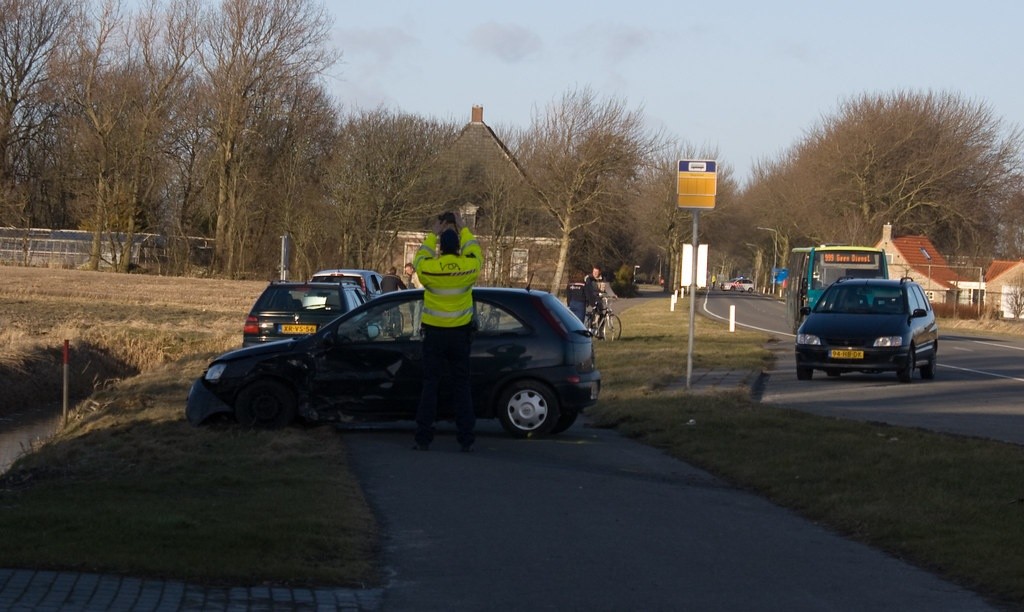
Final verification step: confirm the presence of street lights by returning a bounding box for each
[756,226,777,295]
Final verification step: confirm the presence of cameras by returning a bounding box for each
[438,212,456,223]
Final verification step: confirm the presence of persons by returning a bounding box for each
[565,267,617,339]
[405,263,424,339]
[380,267,407,337]
[414,219,483,451]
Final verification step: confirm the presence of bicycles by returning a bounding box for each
[385,306,404,338]
[476,302,501,331]
[584,293,622,341]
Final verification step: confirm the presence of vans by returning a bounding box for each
[302,270,388,326]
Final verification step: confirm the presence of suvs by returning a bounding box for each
[794,277,939,384]
[241,280,383,352]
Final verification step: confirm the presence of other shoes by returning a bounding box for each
[412,445,430,451]
[462,446,474,452]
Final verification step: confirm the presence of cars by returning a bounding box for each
[184,283,602,439]
[721,277,754,294]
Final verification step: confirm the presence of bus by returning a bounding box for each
[786,244,889,337]
